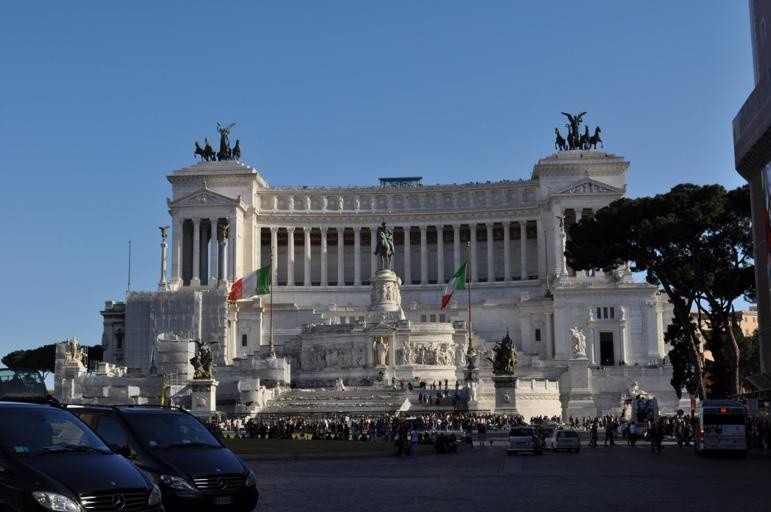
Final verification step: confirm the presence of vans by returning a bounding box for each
[0,400,259,512]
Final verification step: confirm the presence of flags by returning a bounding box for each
[228,265,272,300]
[441,256,468,309]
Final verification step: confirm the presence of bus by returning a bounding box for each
[0,368,49,400]
[693,399,747,457]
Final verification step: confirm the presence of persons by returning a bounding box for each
[193,341,214,381]
[529,411,701,457]
[571,116,583,141]
[505,342,519,374]
[217,126,231,154]
[373,222,396,257]
[209,416,221,441]
[570,326,587,355]
[391,378,469,411]
[746,413,771,452]
[220,413,527,440]
[401,427,461,459]
[63,335,88,366]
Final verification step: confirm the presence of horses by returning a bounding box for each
[233,139,240,160]
[567,125,575,150]
[555,128,565,151]
[588,126,603,150]
[580,126,591,150]
[204,137,213,162]
[194,141,205,161]
[377,231,390,270]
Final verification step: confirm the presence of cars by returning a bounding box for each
[505,426,580,456]
